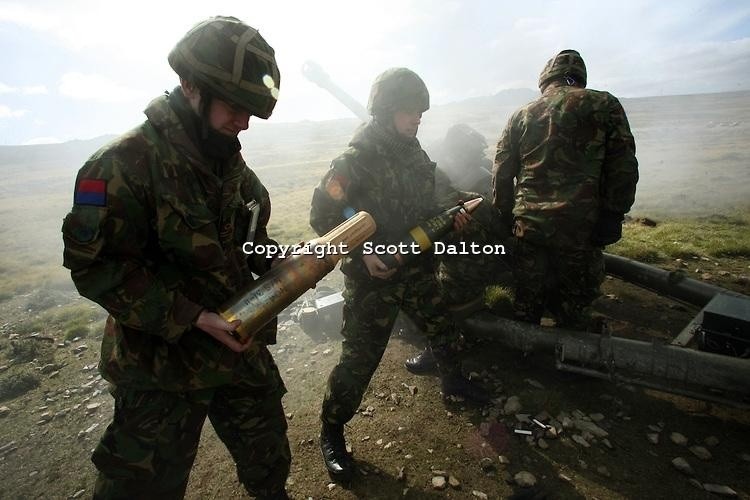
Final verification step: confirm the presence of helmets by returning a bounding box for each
[167,15,281,120]
[539,48,588,89]
[367,66,430,120]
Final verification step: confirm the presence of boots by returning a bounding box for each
[404,334,467,376]
[441,376,489,403]
[320,423,356,476]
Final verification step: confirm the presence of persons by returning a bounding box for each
[60,13,317,500]
[308,66,497,486]
[488,46,639,383]
[400,124,515,372]
